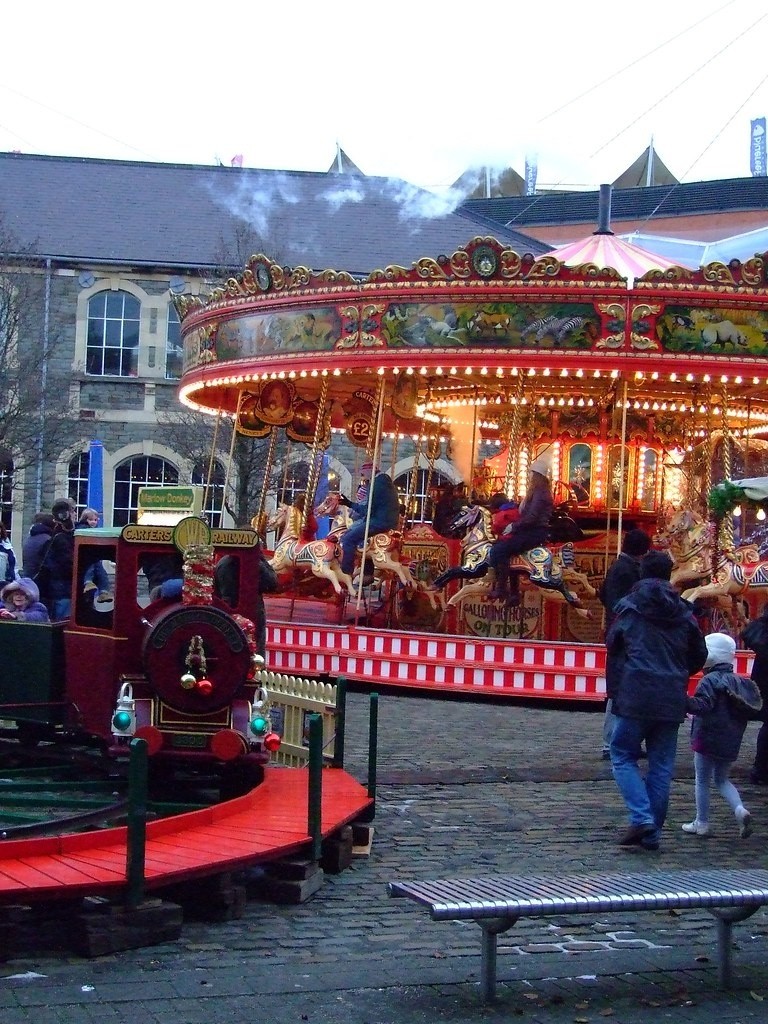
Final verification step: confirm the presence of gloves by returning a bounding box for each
[339,494,352,508]
[501,522,513,536]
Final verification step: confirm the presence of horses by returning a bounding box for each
[251,490,768,628]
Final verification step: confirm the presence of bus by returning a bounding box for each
[1,486,267,795]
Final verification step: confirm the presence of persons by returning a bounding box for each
[605,551,708,851]
[0,577,50,624]
[349,484,368,549]
[338,454,400,575]
[682,632,764,839]
[486,460,554,609]
[23,496,281,670]
[0,520,21,589]
[596,527,650,759]
[738,604,768,789]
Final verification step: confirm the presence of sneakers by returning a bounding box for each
[734,805,752,838]
[681,820,712,836]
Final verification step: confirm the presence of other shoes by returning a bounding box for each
[603,751,647,760]
[491,588,506,597]
[620,824,655,845]
[504,597,520,607]
[643,842,660,850]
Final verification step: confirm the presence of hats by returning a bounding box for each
[53,498,77,531]
[530,461,548,476]
[361,456,378,481]
[704,633,736,668]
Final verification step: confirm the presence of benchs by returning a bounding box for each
[386,869,768,1002]
